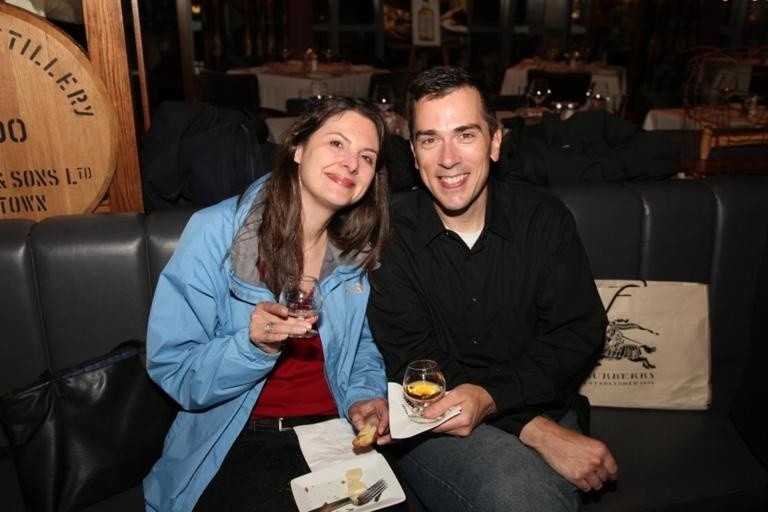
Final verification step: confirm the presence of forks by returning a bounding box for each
[307,479,388,511]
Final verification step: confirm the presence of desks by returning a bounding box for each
[200,51,768,182]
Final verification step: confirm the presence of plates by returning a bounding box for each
[290,453,407,512]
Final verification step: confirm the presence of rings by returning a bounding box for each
[264,322,273,333]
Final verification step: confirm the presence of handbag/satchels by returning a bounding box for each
[572,276,716,414]
[0,338,181,512]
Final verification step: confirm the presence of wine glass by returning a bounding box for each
[278,273,323,339]
[528,79,550,121]
[401,359,446,424]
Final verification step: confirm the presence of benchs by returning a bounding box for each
[0,178,767,512]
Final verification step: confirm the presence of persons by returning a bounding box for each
[140,97,406,512]
[367,66,622,512]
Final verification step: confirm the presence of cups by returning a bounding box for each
[585,81,609,111]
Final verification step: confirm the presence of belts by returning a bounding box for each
[251,414,337,431]
